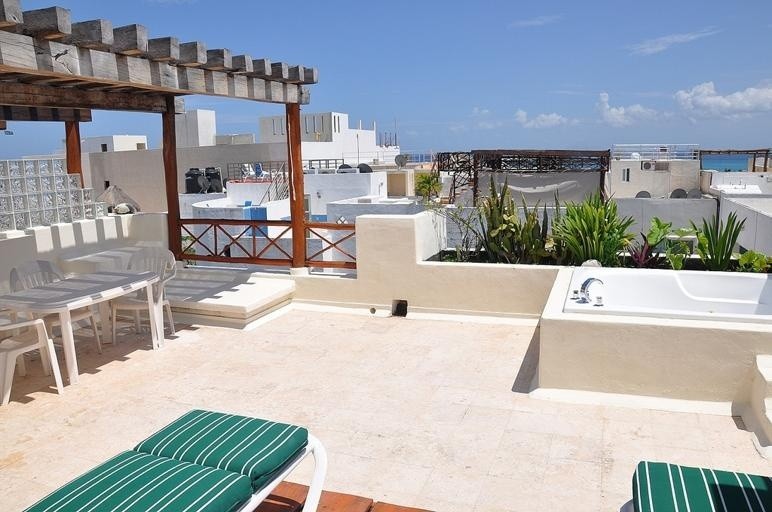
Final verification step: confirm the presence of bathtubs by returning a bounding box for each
[563,266,772,324]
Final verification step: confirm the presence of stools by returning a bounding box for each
[632,459,771,512]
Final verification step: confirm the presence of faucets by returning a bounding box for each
[581,277,605,302]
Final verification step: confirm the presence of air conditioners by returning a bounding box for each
[641,161,656,171]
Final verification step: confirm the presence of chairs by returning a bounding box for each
[0,245,178,406]
[240,163,276,183]
[23,406,328,512]
